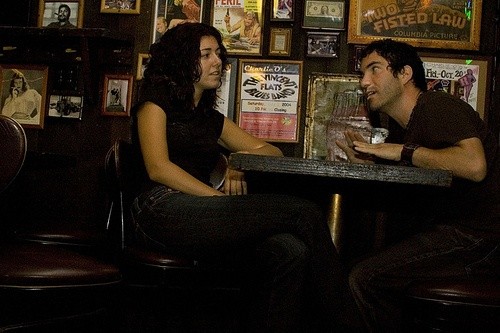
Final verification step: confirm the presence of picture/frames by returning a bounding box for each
[0,0,492,165]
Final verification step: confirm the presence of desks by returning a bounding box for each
[228,153,454,254]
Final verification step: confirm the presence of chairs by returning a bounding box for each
[0,116,198,333]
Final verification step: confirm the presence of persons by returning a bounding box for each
[110,88,120,105]
[2,72,43,125]
[48,4,77,29]
[340,39,500,333]
[121,22,338,333]
[156,17,169,35]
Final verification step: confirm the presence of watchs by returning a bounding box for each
[401,141,421,167]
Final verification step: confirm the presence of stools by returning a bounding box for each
[406,279,500,333]
